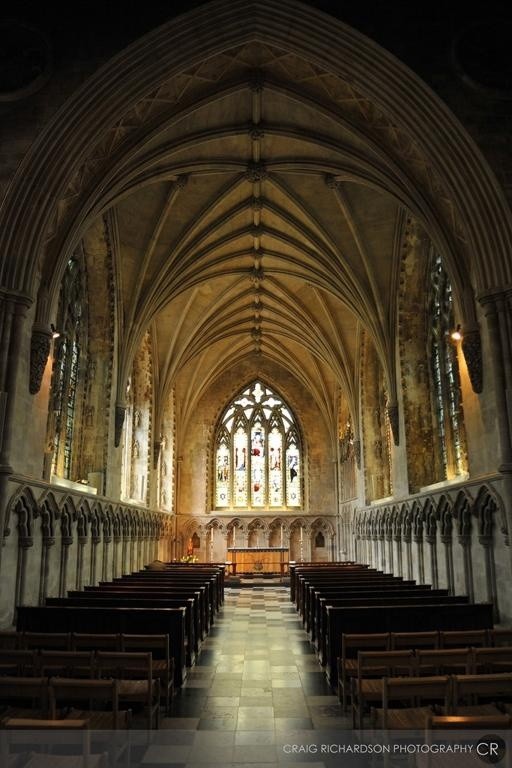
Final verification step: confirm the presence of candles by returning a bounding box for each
[232,525,235,540]
[300,526,302,541]
[280,526,283,539]
[210,528,213,541]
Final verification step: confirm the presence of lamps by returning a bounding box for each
[50,323,59,338]
[451,324,462,340]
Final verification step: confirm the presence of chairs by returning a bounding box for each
[0,631,174,768]
[333,628,511,767]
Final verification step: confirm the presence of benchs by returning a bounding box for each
[287,561,497,697]
[0,564,223,697]
